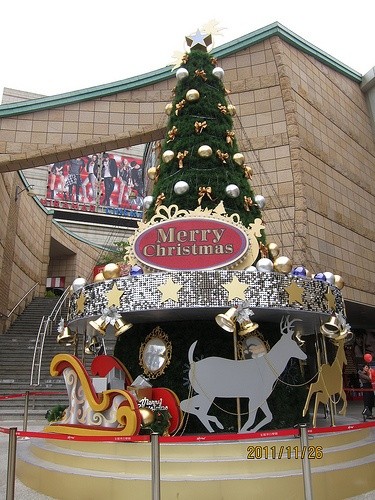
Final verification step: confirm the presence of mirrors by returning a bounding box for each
[139,327,171,379]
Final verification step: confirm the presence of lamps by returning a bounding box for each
[27,186,36,197]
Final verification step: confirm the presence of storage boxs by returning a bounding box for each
[127,385,152,400]
[110,379,124,391]
[90,376,107,394]
[131,375,152,389]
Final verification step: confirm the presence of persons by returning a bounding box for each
[349,372,361,396]
[358,365,375,419]
[49,152,144,211]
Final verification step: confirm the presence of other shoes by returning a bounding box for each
[118,203,122,207]
[63,192,67,200]
[51,190,55,199]
[69,194,72,201]
[76,195,80,204]
[91,200,96,205]
[101,199,106,205]
[85,198,89,203]
[106,199,110,206]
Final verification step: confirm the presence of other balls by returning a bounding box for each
[244,165,253,178]
[246,243,344,291]
[173,181,189,196]
[143,195,154,210]
[93,272,104,282]
[212,67,225,80]
[227,104,236,116]
[225,183,241,199]
[69,285,75,295]
[165,103,174,116]
[175,67,188,80]
[103,263,121,279]
[72,278,87,291]
[197,145,213,158]
[147,167,158,180]
[161,150,174,164]
[233,153,244,166]
[254,195,266,209]
[186,89,200,101]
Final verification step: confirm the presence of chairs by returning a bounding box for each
[50,353,183,437]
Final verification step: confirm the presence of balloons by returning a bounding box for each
[364,354,372,362]
